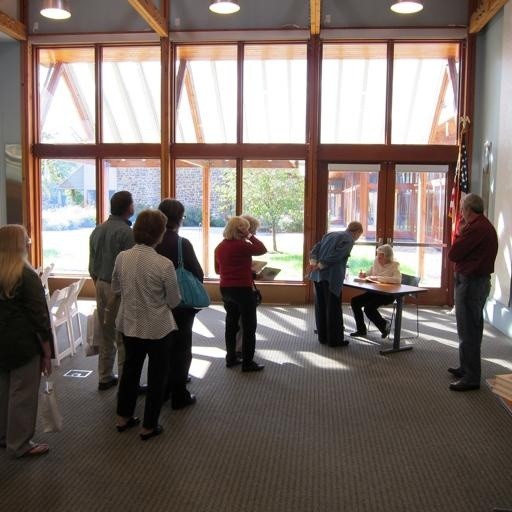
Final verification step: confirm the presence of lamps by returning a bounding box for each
[41,0,71,20]
[208,0,240,14]
[390,0,423,14]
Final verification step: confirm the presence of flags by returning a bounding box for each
[448,130,469,246]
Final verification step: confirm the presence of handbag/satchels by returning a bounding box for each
[85,310,101,357]
[175,269,211,307]
[42,381,62,434]
[252,290,262,306]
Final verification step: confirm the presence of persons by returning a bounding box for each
[0,224,55,461]
[306,221,363,347]
[89,191,147,396]
[235,213,260,358]
[447,193,498,392]
[350,243,401,338]
[215,217,267,373]
[158,199,212,410]
[111,209,182,440]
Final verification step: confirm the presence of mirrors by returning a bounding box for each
[6,144,23,223]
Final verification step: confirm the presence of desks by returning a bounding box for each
[484,378,512,417]
[314,276,429,355]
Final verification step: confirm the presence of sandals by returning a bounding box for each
[16,443,50,455]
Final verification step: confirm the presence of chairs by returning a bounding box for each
[48,283,76,367]
[367,273,420,341]
[51,278,87,354]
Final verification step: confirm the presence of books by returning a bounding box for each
[354,275,382,284]
[253,266,282,281]
[250,260,269,275]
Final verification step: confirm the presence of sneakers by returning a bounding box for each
[337,341,348,347]
[350,331,366,336]
[382,321,391,339]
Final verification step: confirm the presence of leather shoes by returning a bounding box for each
[116,417,138,432]
[226,359,244,368]
[187,377,191,381]
[98,374,118,389]
[139,384,148,395]
[242,364,265,371]
[140,424,163,441]
[448,368,463,377]
[171,394,196,409]
[450,381,480,391]
